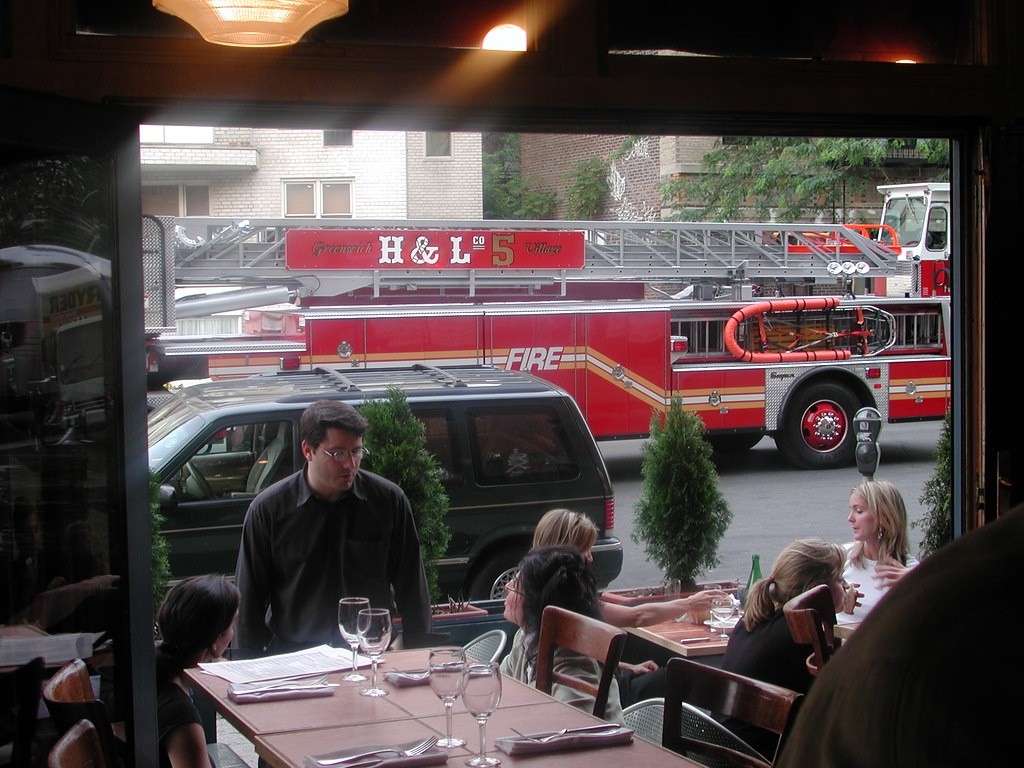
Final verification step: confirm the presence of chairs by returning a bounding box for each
[0,582,844,768]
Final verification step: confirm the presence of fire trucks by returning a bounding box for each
[142,180,952,470]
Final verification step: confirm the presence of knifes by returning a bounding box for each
[495,723,620,741]
[381,667,462,674]
[233,683,340,695]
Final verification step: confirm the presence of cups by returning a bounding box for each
[662,578,681,602]
[703,585,722,591]
[736,576,750,606]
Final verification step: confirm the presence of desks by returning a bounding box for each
[253,699,711,768]
[180,645,557,768]
[621,607,736,657]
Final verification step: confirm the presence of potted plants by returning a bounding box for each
[600,394,744,607]
[359,384,489,628]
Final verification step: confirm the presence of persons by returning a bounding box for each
[155,573,240,768]
[774,501,1024,768]
[498,544,628,729]
[0,473,120,636]
[533,508,731,709]
[835,480,923,624]
[685,536,851,768]
[233,401,432,658]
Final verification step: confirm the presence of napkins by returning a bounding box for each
[494,726,634,757]
[302,737,450,768]
[383,664,491,687]
[228,679,335,703]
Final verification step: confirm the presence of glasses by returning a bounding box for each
[316,445,370,462]
[504,579,524,596]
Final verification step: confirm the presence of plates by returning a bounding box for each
[702,618,741,635]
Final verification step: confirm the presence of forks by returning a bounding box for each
[392,667,490,680]
[254,674,330,696]
[316,735,439,766]
[510,728,620,743]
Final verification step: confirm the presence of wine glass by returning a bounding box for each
[461,662,503,768]
[337,596,372,683]
[711,598,735,641]
[427,646,470,749]
[356,608,392,698]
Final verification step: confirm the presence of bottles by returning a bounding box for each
[744,553,762,611]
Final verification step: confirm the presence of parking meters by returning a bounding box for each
[851,406,884,482]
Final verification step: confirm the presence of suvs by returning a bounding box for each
[147,362,623,631]
